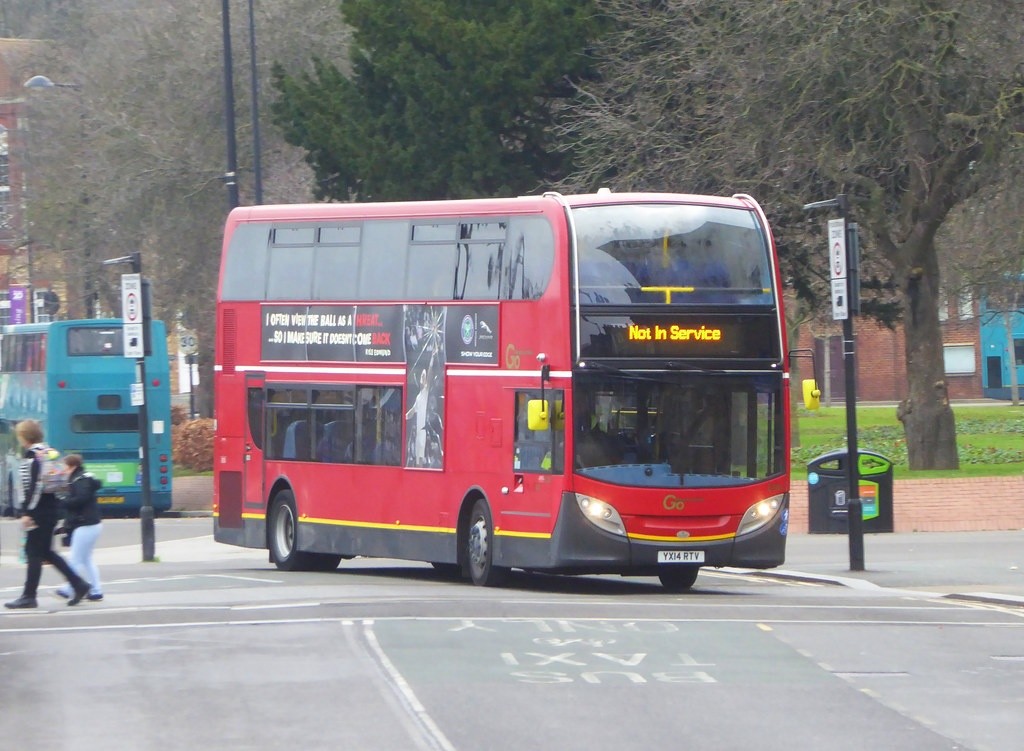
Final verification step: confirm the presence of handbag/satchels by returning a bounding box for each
[18,533,53,565]
[25,445,69,494]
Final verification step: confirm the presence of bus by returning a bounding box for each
[212,188,822,591]
[0,319,173,519]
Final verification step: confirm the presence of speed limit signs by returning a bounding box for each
[177,329,198,355]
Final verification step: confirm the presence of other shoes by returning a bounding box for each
[4,593,38,609]
[68,581,91,606]
[85,592,103,600]
[57,589,70,599]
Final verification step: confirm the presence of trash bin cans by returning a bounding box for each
[806,445,895,534]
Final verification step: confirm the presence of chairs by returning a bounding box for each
[319,420,354,462]
[282,420,310,459]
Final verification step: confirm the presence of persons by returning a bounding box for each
[4,419,102,609]
[406,348,438,466]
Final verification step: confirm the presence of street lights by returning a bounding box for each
[23,75,94,317]
